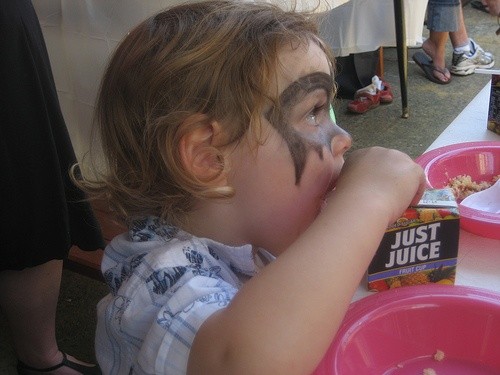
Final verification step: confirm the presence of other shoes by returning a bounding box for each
[17,351,103,375]
[347,82,393,113]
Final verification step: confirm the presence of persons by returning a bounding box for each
[0,1,106,375]
[447,0,495,76]
[92,0,427,375]
[412,0,458,85]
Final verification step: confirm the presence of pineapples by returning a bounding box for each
[400,266,457,285]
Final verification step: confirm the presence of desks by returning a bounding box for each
[313,0,430,120]
[351,80,500,305]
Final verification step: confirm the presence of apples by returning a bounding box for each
[369,280,389,292]
[402,209,455,227]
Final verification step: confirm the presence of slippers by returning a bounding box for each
[412,52,451,83]
[471,0,491,14]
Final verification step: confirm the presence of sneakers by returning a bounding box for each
[450,41,494,75]
[408,36,427,48]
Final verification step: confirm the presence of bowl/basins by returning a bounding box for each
[313,284,500,374]
[415,141,500,242]
